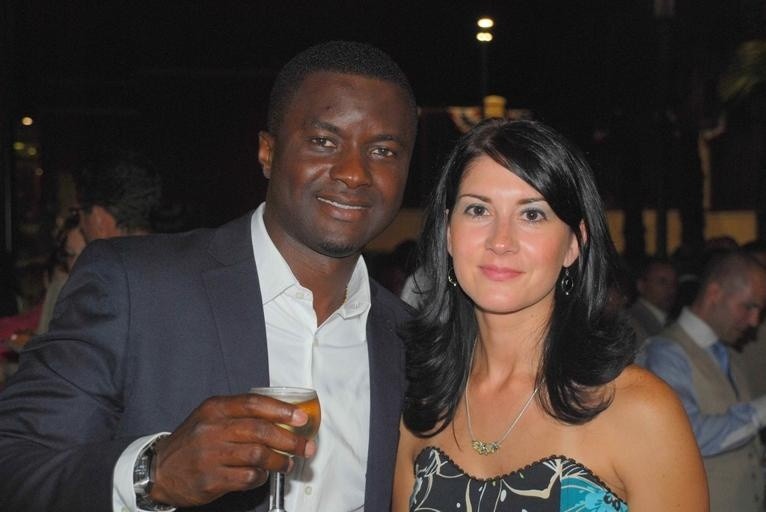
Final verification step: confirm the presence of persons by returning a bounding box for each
[587,239,763,359]
[35,210,87,335]
[2,39,428,511]
[391,117,710,511]
[76,166,170,242]
[367,234,430,297]
[634,251,765,511]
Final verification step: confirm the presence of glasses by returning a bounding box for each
[68,204,90,216]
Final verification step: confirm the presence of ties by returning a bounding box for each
[712,340,730,373]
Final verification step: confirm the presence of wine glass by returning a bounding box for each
[249,386,320,512]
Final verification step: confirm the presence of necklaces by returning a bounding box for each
[466,328,549,454]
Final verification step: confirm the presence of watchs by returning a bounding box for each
[134,433,173,512]
